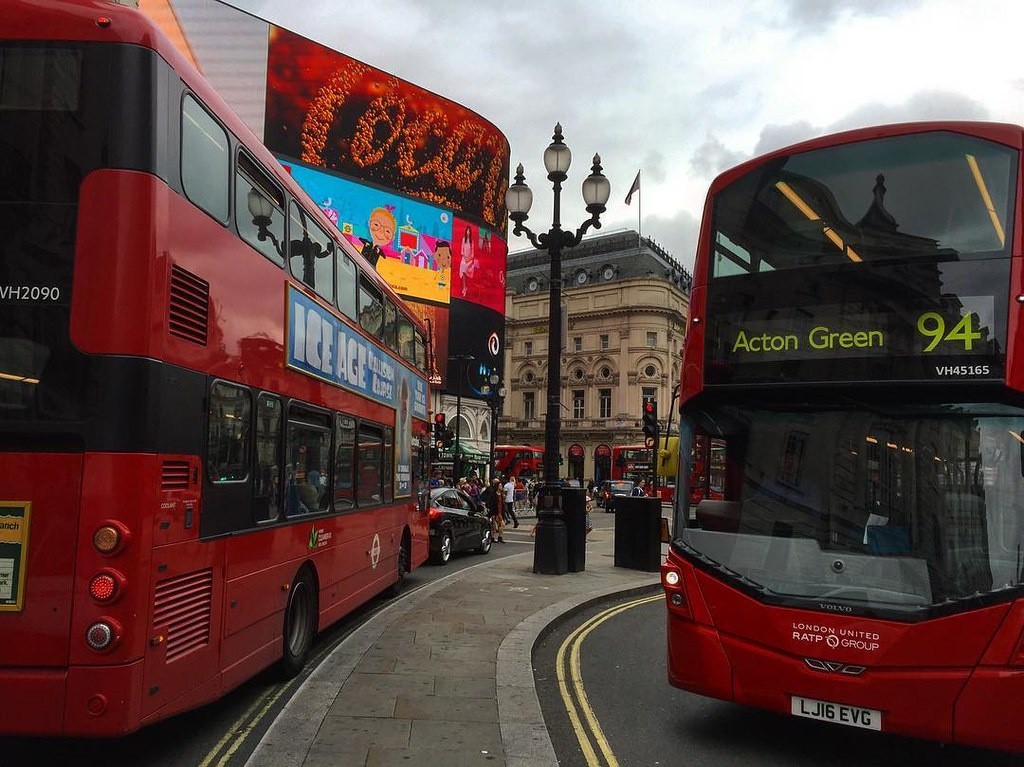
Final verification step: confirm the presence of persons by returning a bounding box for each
[586,495,593,542]
[559,478,580,487]
[430,469,519,544]
[632,477,653,497]
[514,476,545,508]
[459,226,475,297]
[587,478,605,499]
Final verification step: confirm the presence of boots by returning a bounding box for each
[498,536,505,544]
[492,537,498,543]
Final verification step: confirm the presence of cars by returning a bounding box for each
[595,479,635,514]
[428,487,493,565]
[563,477,582,488]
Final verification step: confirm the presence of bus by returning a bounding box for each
[656,119,1024,755]
[494,444,546,485]
[1,0,436,745]
[610,435,727,505]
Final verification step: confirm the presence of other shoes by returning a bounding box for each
[513,522,519,528]
[506,520,510,523]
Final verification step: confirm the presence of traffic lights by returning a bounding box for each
[642,395,660,449]
[434,413,446,442]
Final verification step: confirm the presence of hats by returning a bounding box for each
[586,495,592,502]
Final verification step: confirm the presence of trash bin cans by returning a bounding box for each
[614,494,663,572]
[537,487,587,572]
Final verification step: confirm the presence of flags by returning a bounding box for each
[624,172,639,205]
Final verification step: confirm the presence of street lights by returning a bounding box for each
[447,354,474,486]
[505,120,610,574]
